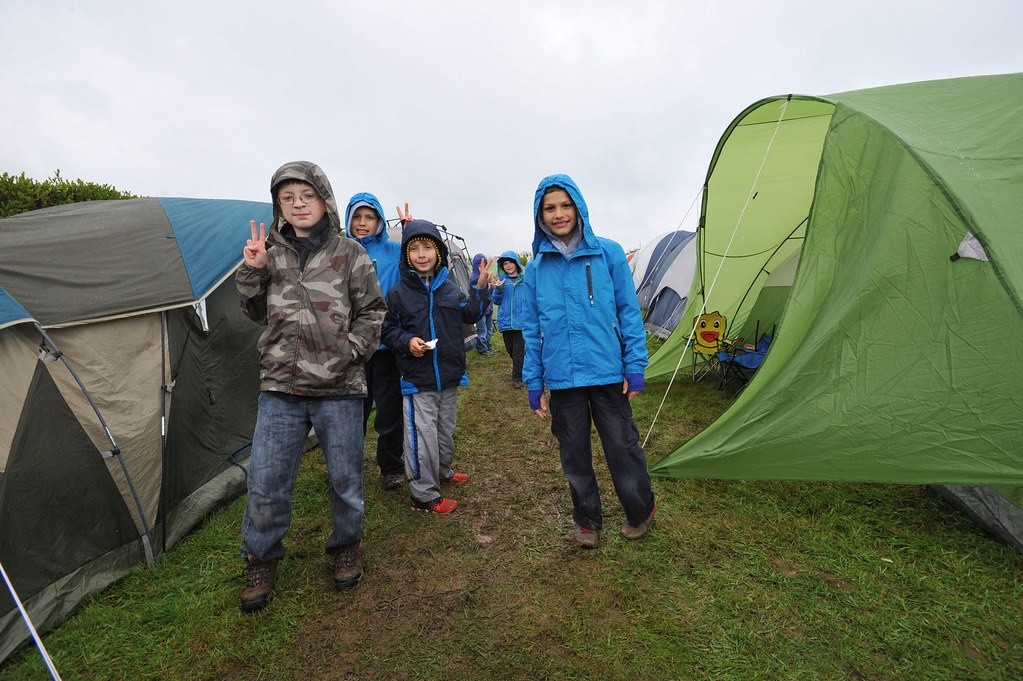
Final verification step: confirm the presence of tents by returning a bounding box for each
[633,73,1023,485]
[0,201,322,664]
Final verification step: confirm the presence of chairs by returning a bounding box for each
[682,311,776,401]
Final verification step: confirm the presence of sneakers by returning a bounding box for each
[332,536,366,589]
[387,474,406,489]
[240,553,277,612]
[410,493,458,515]
[620,502,657,540]
[573,525,599,550]
[440,471,469,484]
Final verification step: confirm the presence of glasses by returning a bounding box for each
[277,190,319,206]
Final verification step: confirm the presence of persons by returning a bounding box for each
[382,219,494,513]
[468,253,495,356]
[345,192,414,488]
[235,161,386,611]
[491,251,526,389]
[521,174,657,550]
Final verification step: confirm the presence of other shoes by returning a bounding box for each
[513,380,523,389]
[481,348,497,355]
